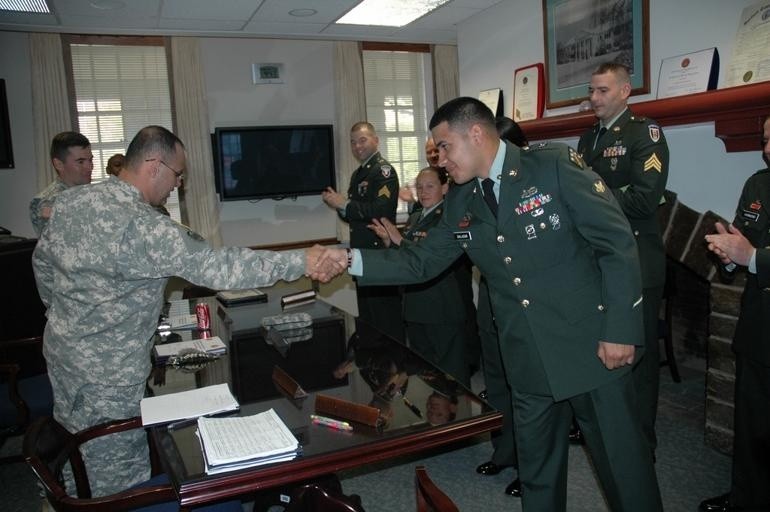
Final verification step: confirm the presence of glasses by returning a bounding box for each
[146,159,183,181]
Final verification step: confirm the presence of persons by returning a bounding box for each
[571,61,673,470]
[399,138,450,215]
[105,154,127,177]
[30,130,93,237]
[697,114,770,512]
[476,117,529,497]
[323,122,401,331]
[368,168,474,388]
[310,96,663,510]
[405,352,466,426]
[30,127,340,512]
[334,320,409,432]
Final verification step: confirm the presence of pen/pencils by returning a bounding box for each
[313,419,352,430]
[310,414,350,426]
[168,414,211,429]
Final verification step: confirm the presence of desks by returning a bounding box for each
[150,293,508,505]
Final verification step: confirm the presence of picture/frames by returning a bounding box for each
[543,0,651,110]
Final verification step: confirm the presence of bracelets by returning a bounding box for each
[346,247,351,268]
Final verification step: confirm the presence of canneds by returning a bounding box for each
[199,331,212,339]
[195,303,211,330]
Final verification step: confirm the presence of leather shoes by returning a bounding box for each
[477,462,517,474]
[506,477,522,497]
[699,490,770,512]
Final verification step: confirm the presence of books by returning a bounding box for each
[214,289,268,306]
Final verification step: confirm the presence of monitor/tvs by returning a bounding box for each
[210,124,337,203]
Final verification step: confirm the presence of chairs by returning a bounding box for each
[22,409,246,511]
[1,338,54,465]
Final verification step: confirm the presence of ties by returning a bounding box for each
[482,178,498,220]
[595,128,606,150]
